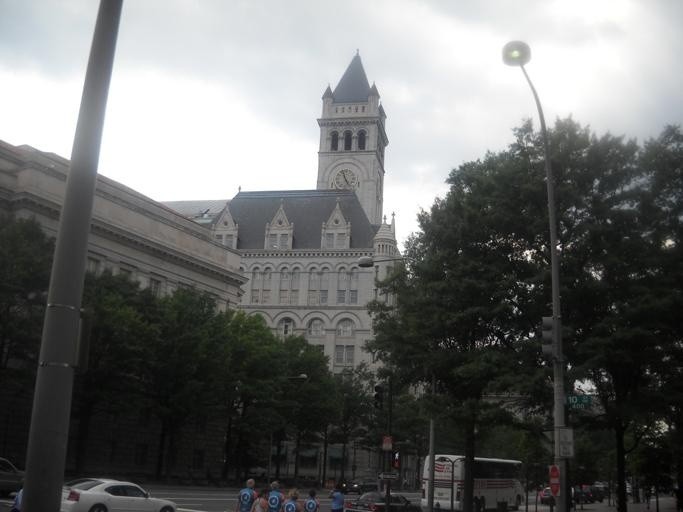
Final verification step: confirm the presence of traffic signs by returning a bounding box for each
[376,472,399,480]
[566,394,591,411]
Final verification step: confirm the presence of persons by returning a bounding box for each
[327,484,344,512]
[7,477,24,511]
[231,478,321,512]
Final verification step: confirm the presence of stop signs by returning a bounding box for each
[548,463,561,498]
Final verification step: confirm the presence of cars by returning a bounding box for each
[60,475,178,512]
[0,455,25,496]
[539,481,658,506]
[340,475,377,495]
[341,490,418,512]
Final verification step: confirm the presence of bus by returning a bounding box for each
[418,454,525,511]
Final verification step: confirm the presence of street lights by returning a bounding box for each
[267,374,309,482]
[356,254,437,511]
[503,40,569,511]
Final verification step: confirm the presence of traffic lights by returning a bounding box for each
[372,382,386,409]
[393,450,402,469]
[540,315,555,361]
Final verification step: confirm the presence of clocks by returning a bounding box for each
[333,168,358,190]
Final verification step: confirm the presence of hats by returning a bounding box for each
[247,479,255,488]
